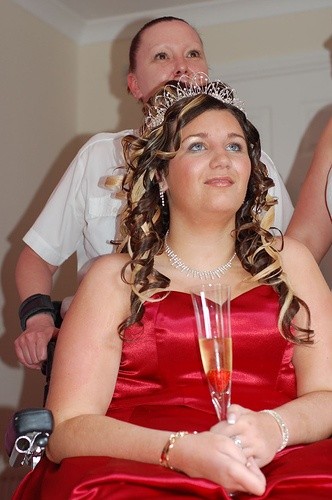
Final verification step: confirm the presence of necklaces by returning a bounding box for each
[163,227,240,281]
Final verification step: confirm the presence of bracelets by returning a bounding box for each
[261,410,289,453]
[18,293,54,331]
[160,430,189,471]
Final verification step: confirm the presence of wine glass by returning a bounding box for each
[186,282,232,422]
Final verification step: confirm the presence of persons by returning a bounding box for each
[284,117,332,266]
[13,15,297,369]
[11,72,332,500]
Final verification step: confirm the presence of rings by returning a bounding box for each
[233,435,242,447]
